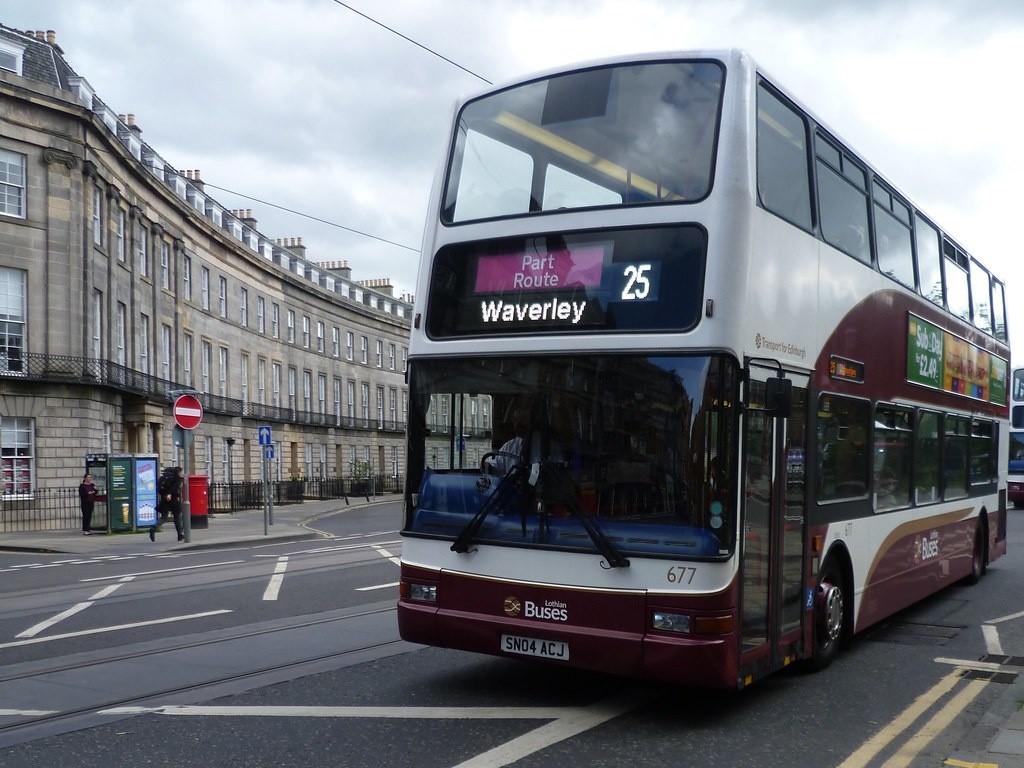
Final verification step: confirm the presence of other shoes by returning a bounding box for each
[149,529,154,542]
[178,535,184,541]
[84,530,94,535]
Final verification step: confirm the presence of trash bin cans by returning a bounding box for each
[359,477,371,493]
[342,479,351,494]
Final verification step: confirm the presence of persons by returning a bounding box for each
[150,467,184,542]
[478,402,896,510]
[79,473,98,536]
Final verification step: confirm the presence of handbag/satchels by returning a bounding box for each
[155,501,169,514]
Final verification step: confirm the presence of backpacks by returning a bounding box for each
[159,467,175,494]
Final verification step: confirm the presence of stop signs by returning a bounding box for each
[173,394,203,430]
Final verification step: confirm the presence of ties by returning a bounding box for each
[519,442,527,462]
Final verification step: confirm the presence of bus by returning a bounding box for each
[396,47,1014,692]
[785,422,993,512]
[1007,366,1024,511]
